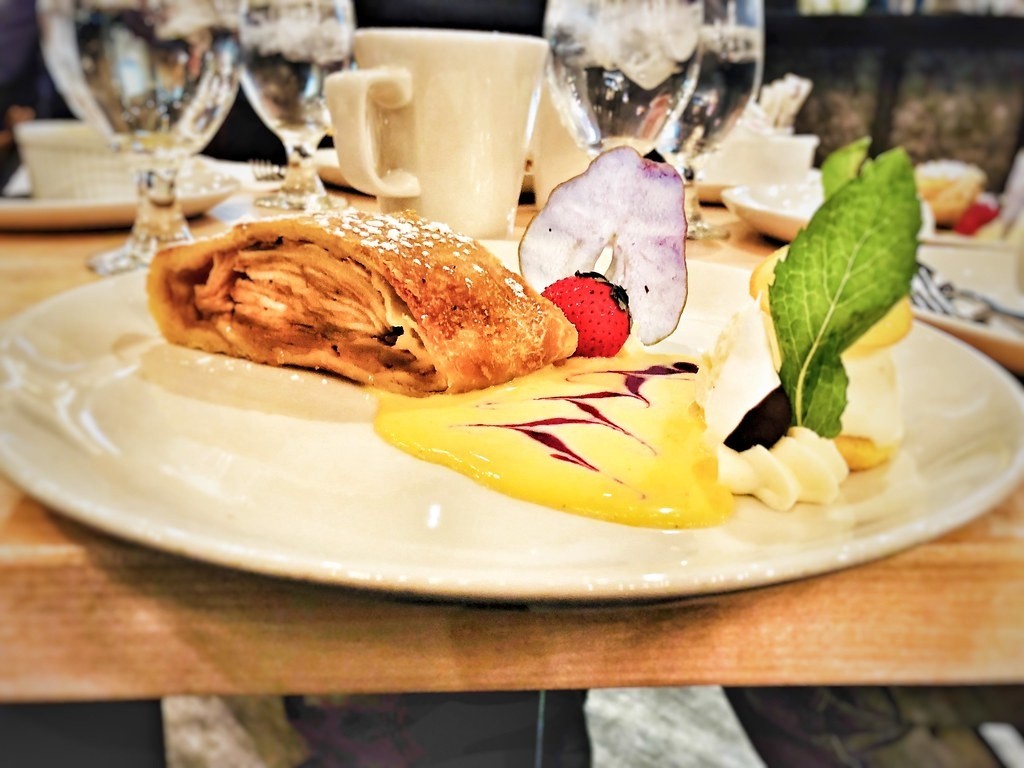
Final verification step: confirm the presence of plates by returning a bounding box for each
[2,237,1023,601]
[721,185,1024,244]
[907,244,1024,374]
[1,161,240,229]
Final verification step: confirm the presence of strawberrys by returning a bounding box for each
[957,195,1001,235]
[540,271,633,357]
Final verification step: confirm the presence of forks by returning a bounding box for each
[252,160,285,181]
[909,267,955,316]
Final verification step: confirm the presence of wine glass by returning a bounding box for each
[655,0,764,239]
[38,0,240,277]
[240,1,355,213]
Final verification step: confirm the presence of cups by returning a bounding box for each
[18,120,125,198]
[324,28,548,239]
[526,78,592,211]
[542,0,702,162]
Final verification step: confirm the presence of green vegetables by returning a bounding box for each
[764,134,921,439]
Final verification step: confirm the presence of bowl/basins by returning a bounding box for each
[701,135,817,204]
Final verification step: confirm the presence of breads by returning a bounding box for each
[908,159,988,226]
[145,210,577,397]
